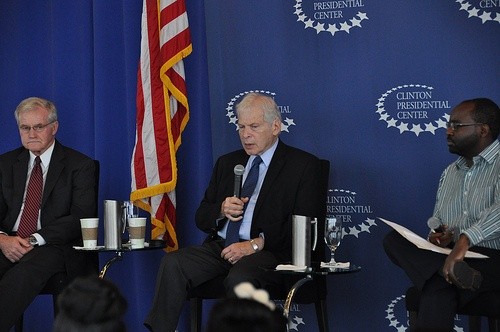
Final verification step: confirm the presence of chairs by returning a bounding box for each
[191,159,362,332]
[405,286,500,332]
[14,160,167,332]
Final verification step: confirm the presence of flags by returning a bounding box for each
[130,1,192,252]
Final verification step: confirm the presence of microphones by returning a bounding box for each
[427,217,445,234]
[233,165,245,198]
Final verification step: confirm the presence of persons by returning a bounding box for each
[383,98,500,332]
[55,275,125,332]
[145,93,329,332]
[0,97,97,332]
[208,297,288,332]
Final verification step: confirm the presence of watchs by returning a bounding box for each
[29,235,38,248]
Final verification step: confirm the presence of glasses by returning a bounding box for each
[446,121,482,130]
[18,120,56,132]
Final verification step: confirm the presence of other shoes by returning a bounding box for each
[449,259,483,292]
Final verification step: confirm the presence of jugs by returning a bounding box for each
[103,200,127,250]
[294,215,318,270]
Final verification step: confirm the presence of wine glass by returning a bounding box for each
[322,216,343,268]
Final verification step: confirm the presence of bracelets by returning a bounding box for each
[250,240,259,252]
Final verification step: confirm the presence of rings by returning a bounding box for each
[230,259,233,262]
[437,240,440,244]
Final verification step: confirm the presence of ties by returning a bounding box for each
[16,156,43,240]
[224,156,264,248]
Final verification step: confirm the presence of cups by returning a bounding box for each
[127,218,147,249]
[80,218,100,247]
